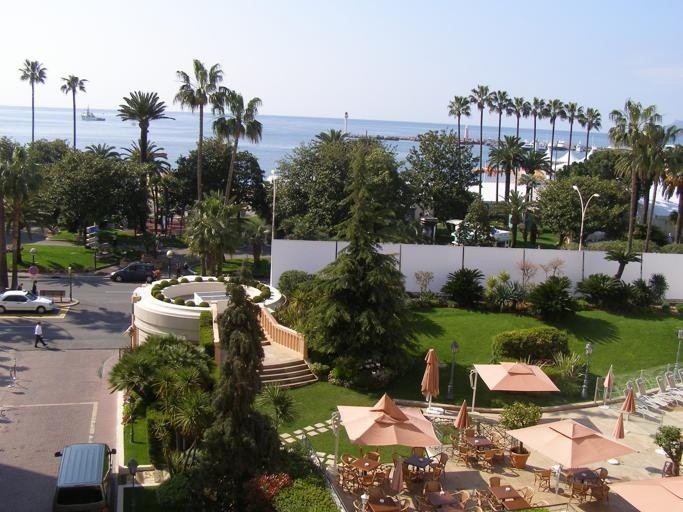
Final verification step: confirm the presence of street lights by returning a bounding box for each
[127,459,138,512]
[673,329,683,387]
[581,342,593,399]
[166,249,175,277]
[571,185,599,251]
[29,249,38,267]
[445,342,461,400]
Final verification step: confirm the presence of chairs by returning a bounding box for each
[626,367,683,423]
[594,377,616,407]
[336,428,610,512]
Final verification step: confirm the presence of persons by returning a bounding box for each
[33,280,37,295]
[35,322,48,348]
[19,282,24,290]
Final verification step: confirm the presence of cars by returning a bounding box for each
[0,290,55,314]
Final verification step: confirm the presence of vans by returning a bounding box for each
[110,261,157,284]
[51,443,116,512]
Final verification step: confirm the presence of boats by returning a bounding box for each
[81,107,107,122]
